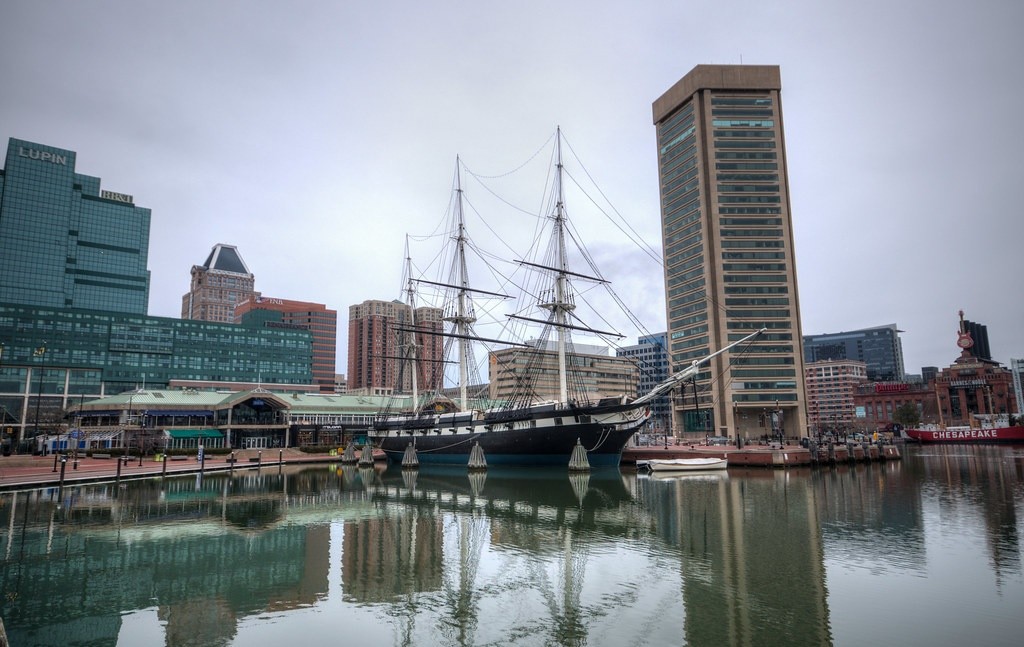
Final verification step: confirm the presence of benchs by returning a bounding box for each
[171,456,188,460]
[196,455,211,459]
[76,452,86,458]
[121,455,136,460]
[92,453,111,459]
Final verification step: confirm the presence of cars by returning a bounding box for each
[640,432,885,448]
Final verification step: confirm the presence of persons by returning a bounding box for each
[873,431,878,440]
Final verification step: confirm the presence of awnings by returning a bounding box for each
[163,428,224,440]
[161,489,220,501]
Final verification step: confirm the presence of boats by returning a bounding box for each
[904,384,1024,442]
[635,455,729,471]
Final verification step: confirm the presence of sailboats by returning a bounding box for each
[367,125,768,471]
